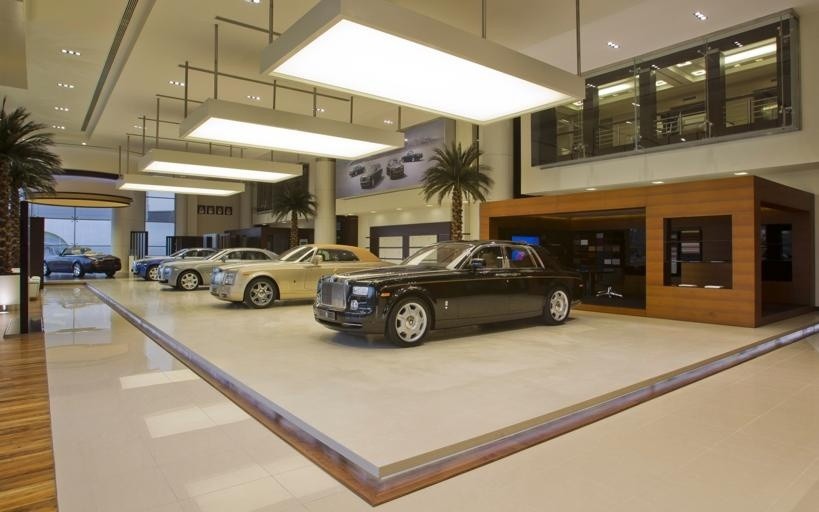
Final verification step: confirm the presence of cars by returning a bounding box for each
[313,239,583,349]
[131,248,220,282]
[210,243,398,309]
[349,163,382,189]
[386,150,423,180]
[157,247,280,291]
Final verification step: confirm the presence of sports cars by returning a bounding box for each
[42,245,122,278]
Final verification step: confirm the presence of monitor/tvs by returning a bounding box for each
[509,234,542,262]
